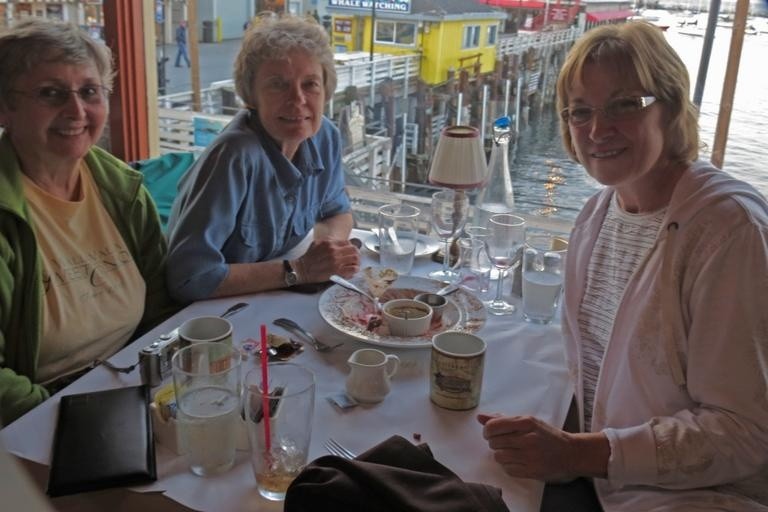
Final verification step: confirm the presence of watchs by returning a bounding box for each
[282,258,300,289]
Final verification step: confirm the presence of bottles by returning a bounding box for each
[472,126,514,281]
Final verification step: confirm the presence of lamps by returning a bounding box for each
[428,124,489,265]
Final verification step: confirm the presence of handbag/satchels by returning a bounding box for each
[45,384,157,497]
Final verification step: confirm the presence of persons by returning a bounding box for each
[245,19,252,30]
[165,13,363,304]
[478,18,768,511]
[0,19,168,427]
[175,20,191,67]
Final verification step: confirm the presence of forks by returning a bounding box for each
[272,317,344,352]
[323,437,356,460]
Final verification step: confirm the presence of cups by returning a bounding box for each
[427,331,488,411]
[244,361,316,502]
[377,204,421,273]
[172,343,243,478]
[520,233,568,326]
[345,348,401,405]
[177,315,233,385]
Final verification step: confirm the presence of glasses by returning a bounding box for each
[13,84,112,107]
[560,95,656,127]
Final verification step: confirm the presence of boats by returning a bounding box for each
[642,4,768,48]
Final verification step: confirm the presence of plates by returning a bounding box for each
[365,230,437,259]
[319,272,488,349]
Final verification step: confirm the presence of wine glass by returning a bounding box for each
[482,214,527,316]
[428,191,469,287]
